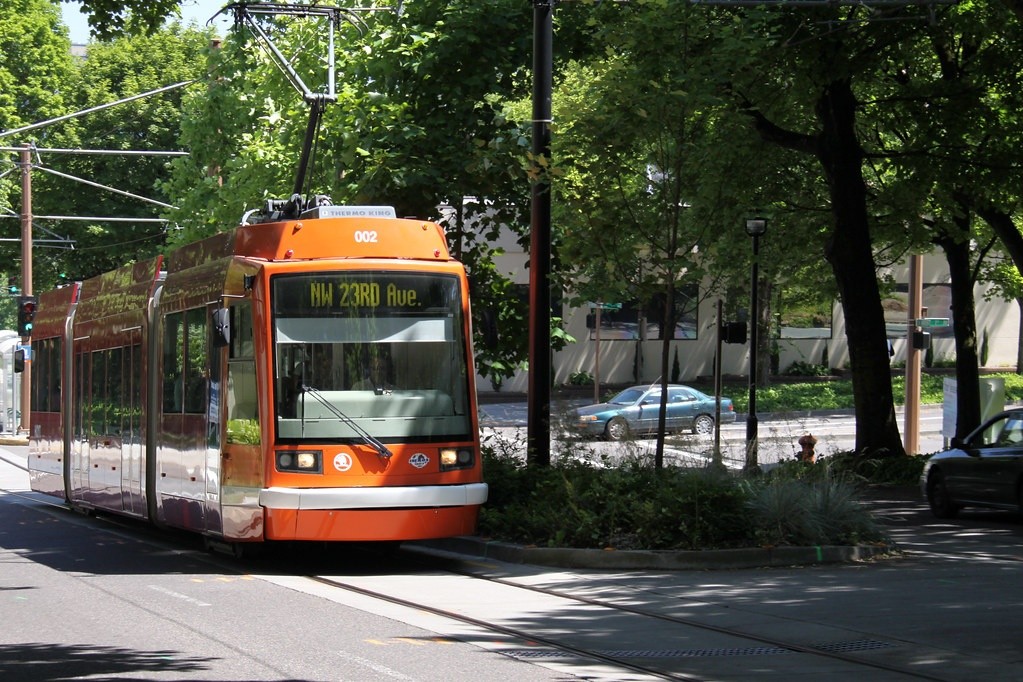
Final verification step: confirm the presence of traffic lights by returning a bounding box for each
[18,296,35,335]
[7,266,19,294]
[57,260,67,282]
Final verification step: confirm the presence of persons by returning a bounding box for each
[174,363,200,411]
[349,354,401,390]
[295,344,326,391]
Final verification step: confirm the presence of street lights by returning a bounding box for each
[742,216,767,473]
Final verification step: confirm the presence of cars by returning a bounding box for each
[920,408,1023,526]
[562,383,737,441]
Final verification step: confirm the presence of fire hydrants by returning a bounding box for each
[795,434,817,462]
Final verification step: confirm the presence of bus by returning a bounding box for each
[27,205,489,562]
